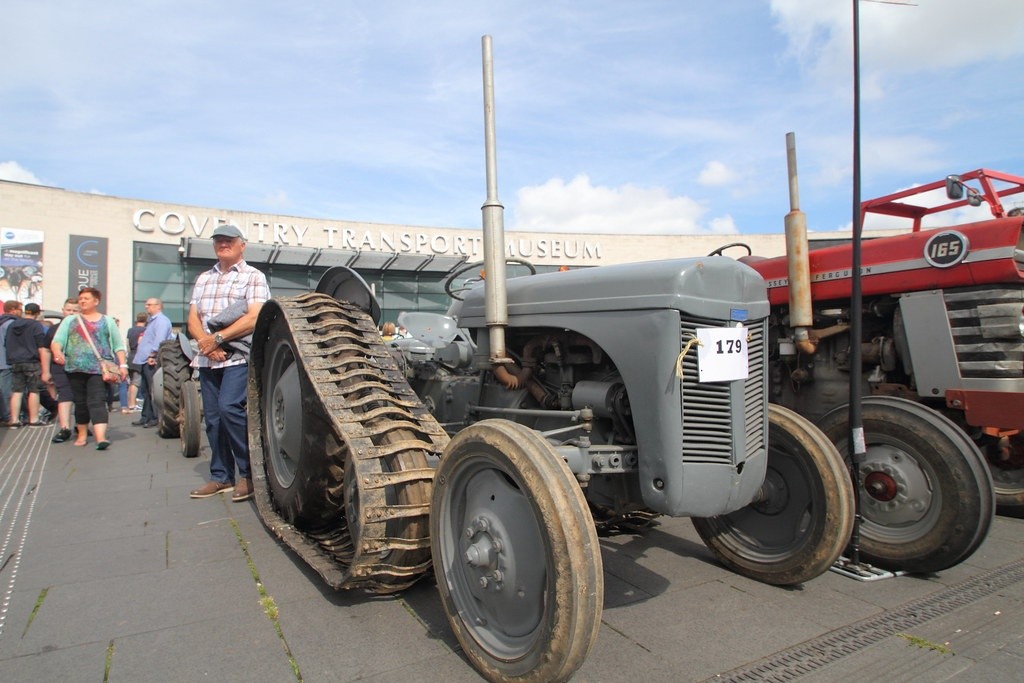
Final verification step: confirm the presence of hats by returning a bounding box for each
[210,224,245,242]
[25,303,44,315]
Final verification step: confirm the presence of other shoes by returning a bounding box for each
[143,418,158,427]
[131,417,149,425]
[121,405,143,414]
[96,440,111,450]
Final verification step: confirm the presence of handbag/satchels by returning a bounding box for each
[99,359,122,385]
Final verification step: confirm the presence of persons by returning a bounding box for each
[5,303,53,429]
[188,225,271,501]
[52,287,129,450]
[107,312,148,414]
[0,300,23,426]
[132,298,172,427]
[382,321,396,341]
[43,298,92,442]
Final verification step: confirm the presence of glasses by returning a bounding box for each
[145,303,159,306]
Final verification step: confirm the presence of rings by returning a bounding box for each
[56,358,58,360]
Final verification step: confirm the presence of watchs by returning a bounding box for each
[215,332,223,344]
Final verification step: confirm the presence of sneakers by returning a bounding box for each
[190,480,234,497]
[74,426,93,435]
[52,426,72,442]
[232,476,255,501]
[7,421,28,429]
[28,420,54,427]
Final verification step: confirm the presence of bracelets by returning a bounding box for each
[120,364,129,368]
[149,356,154,358]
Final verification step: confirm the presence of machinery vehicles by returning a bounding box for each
[247,34,859,683]
[708,133,1024,575]
[151,334,209,459]
